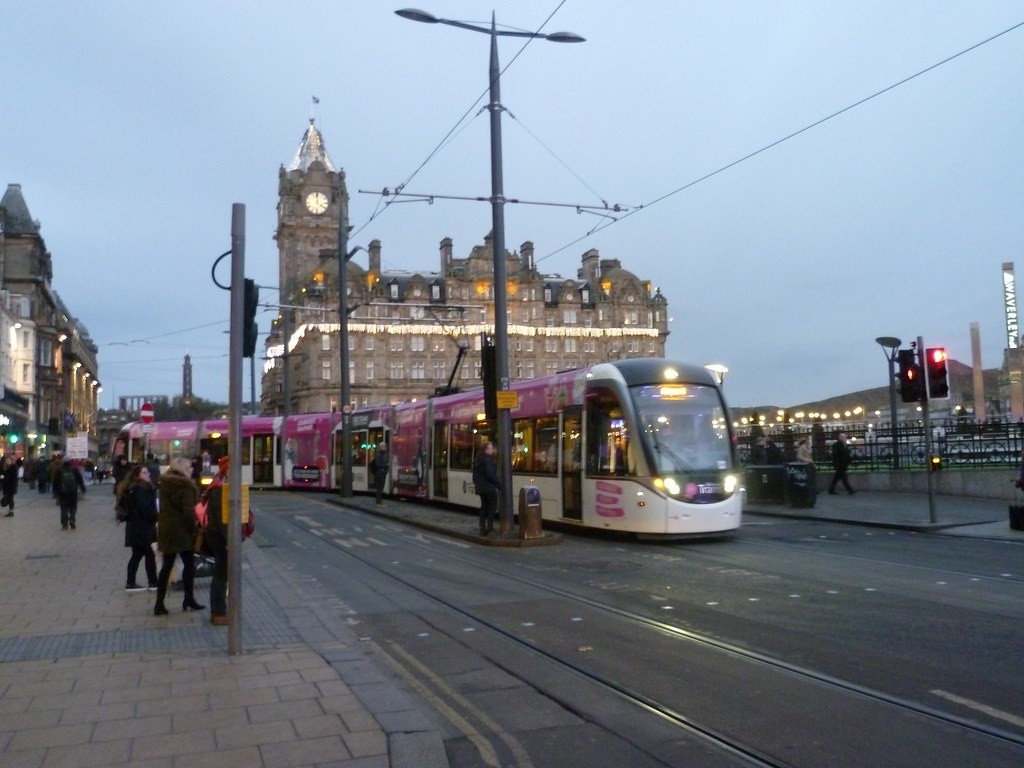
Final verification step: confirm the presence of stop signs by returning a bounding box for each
[140,403,155,424]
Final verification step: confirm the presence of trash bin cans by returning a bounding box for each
[785,462,816,508]
[745,466,788,507]
[518,487,545,539]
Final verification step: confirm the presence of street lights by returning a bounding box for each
[875,336,902,470]
[395,8,588,534]
[222,328,284,415]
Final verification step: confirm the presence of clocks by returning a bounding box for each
[304,191,329,217]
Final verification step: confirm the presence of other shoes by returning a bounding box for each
[70,521,76,528]
[63,524,67,528]
[149,583,158,591]
[480,530,487,536]
[125,583,146,592]
[5,512,13,517]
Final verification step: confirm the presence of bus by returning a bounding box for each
[109,305,744,541]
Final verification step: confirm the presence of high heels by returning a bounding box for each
[183,599,205,610]
[155,602,169,615]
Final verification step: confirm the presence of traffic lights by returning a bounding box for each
[923,346,951,402]
[243,278,261,358]
[903,363,921,393]
[893,349,919,403]
[8,424,19,445]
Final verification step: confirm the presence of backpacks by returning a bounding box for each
[115,490,134,522]
[59,467,76,492]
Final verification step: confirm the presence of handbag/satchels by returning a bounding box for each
[194,528,211,555]
[369,459,377,474]
[195,503,208,527]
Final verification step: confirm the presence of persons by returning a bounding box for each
[116,466,159,591]
[375,442,391,507]
[155,458,204,615]
[114,457,128,491]
[202,457,229,624]
[751,437,766,465]
[192,452,211,478]
[0,453,22,517]
[24,455,94,529]
[766,438,784,464]
[828,433,857,495]
[796,439,813,462]
[97,457,104,483]
[1015,449,1024,492]
[472,441,501,532]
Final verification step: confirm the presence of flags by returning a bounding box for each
[313,97,319,104]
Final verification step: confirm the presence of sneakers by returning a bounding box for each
[210,614,228,625]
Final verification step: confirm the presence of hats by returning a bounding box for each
[219,456,230,474]
